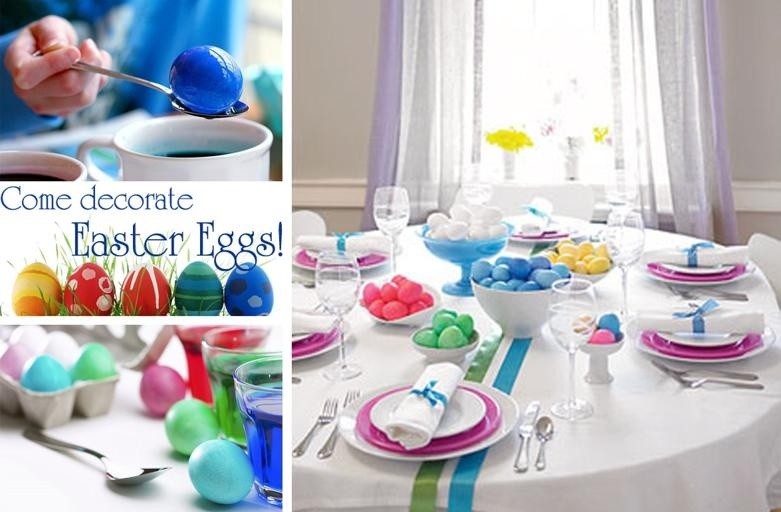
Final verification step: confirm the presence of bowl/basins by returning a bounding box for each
[472,270,574,338]
[408,325,481,359]
[566,263,612,293]
[376,281,440,328]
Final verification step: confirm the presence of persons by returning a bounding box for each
[0,2,247,182]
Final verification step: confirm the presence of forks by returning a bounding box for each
[652,364,767,390]
[666,282,750,303]
[291,389,361,466]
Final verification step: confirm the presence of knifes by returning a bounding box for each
[512,399,541,473]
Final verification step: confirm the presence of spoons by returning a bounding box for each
[534,417,556,470]
[22,425,167,490]
[32,49,251,120]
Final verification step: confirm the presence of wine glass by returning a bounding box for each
[547,278,596,421]
[371,185,409,278]
[416,219,513,298]
[604,212,645,323]
[580,333,627,386]
[313,250,361,382]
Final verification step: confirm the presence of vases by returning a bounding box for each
[502,147,516,180]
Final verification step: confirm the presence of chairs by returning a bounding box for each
[742,228,781,302]
[295,208,325,233]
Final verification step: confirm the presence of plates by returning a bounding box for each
[295,317,352,364]
[292,241,389,273]
[505,219,578,244]
[642,255,758,286]
[337,376,520,465]
[632,322,778,365]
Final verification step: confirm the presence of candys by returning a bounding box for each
[362,204,620,349]
[165,397,219,456]
[1,326,117,393]
[188,438,255,505]
[168,45,244,115]
[139,365,186,417]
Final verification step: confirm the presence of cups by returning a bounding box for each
[202,326,281,453]
[175,324,271,409]
[0,148,87,181]
[233,357,283,503]
[75,111,275,182]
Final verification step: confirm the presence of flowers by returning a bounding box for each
[592,127,615,149]
[487,122,534,152]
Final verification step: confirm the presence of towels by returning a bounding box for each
[299,234,404,262]
[520,195,557,234]
[386,360,463,451]
[649,243,754,269]
[626,309,767,339]
